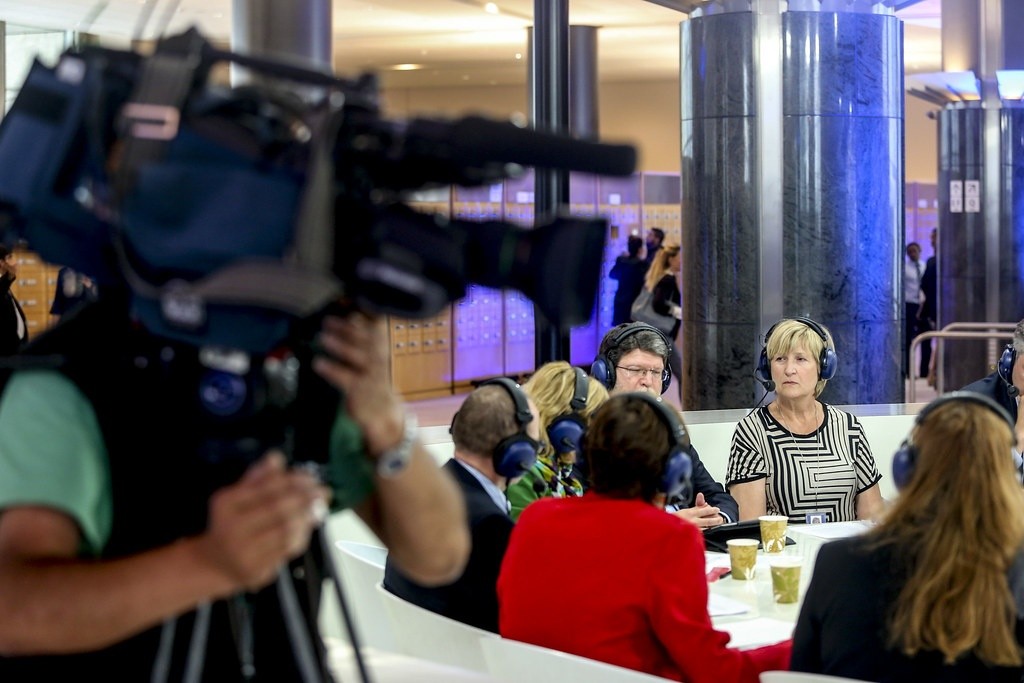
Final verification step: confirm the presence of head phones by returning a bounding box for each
[757,316,838,380]
[481,376,539,479]
[892,391,1019,492]
[545,366,590,454]
[998,344,1017,377]
[590,324,672,395]
[623,390,693,497]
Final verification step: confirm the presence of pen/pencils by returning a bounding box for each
[720,571,731,579]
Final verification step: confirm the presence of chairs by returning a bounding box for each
[325,409,936,682]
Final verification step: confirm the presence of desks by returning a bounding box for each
[701,515,884,654]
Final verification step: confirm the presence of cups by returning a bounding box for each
[759,515,789,554]
[727,539,760,579]
[769,556,802,604]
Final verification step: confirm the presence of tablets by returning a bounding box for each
[701,519,762,534]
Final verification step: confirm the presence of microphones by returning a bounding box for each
[997,363,1020,396]
[405,116,638,177]
[754,368,776,392]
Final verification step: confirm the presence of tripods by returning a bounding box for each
[151,443,371,683]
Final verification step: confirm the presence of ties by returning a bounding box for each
[916,263,921,282]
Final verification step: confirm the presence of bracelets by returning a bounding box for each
[375,409,417,484]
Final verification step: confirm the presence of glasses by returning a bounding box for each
[537,441,546,455]
[615,365,669,381]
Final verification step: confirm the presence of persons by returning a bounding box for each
[382,378,538,633]
[598,321,739,532]
[920,229,937,331]
[503,361,610,523]
[905,242,932,378]
[497,391,793,683]
[951,318,1024,477]
[789,393,1024,683]
[1,286,468,682]
[607,227,683,406]
[726,317,887,522]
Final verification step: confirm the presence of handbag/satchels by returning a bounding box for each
[631,274,676,335]
